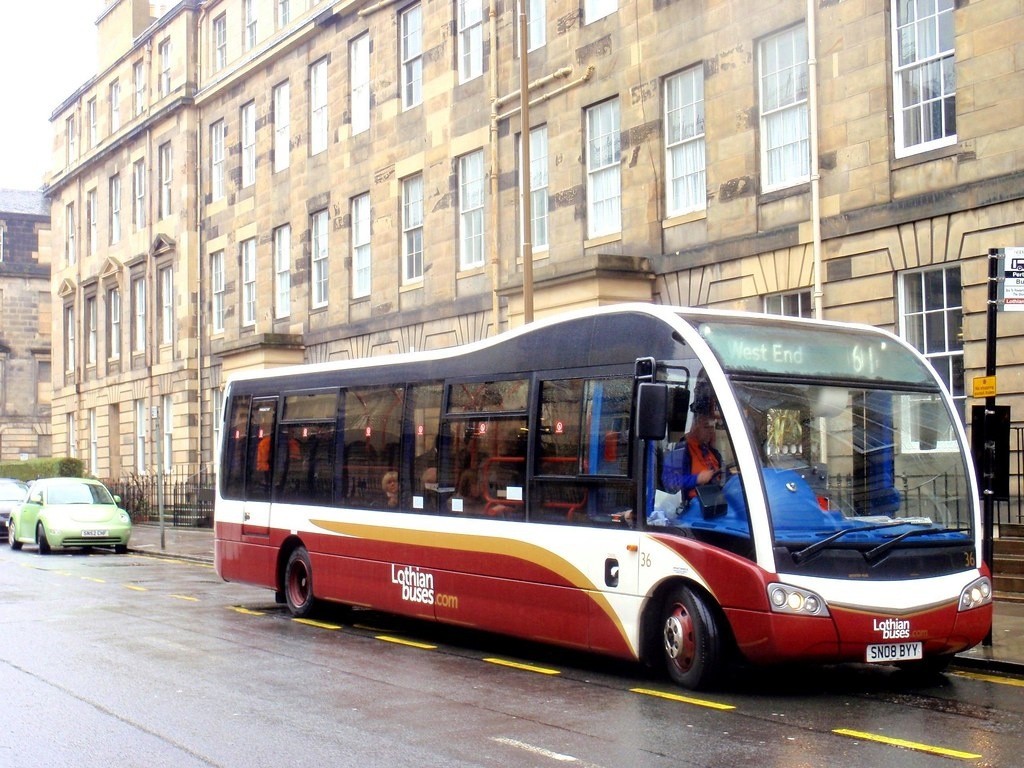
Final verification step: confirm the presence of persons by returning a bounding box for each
[661,410,731,493]
[370,463,488,514]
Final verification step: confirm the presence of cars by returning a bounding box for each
[7,477,131,554]
[0,478,30,536]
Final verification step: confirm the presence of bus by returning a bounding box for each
[215,302,994,692]
